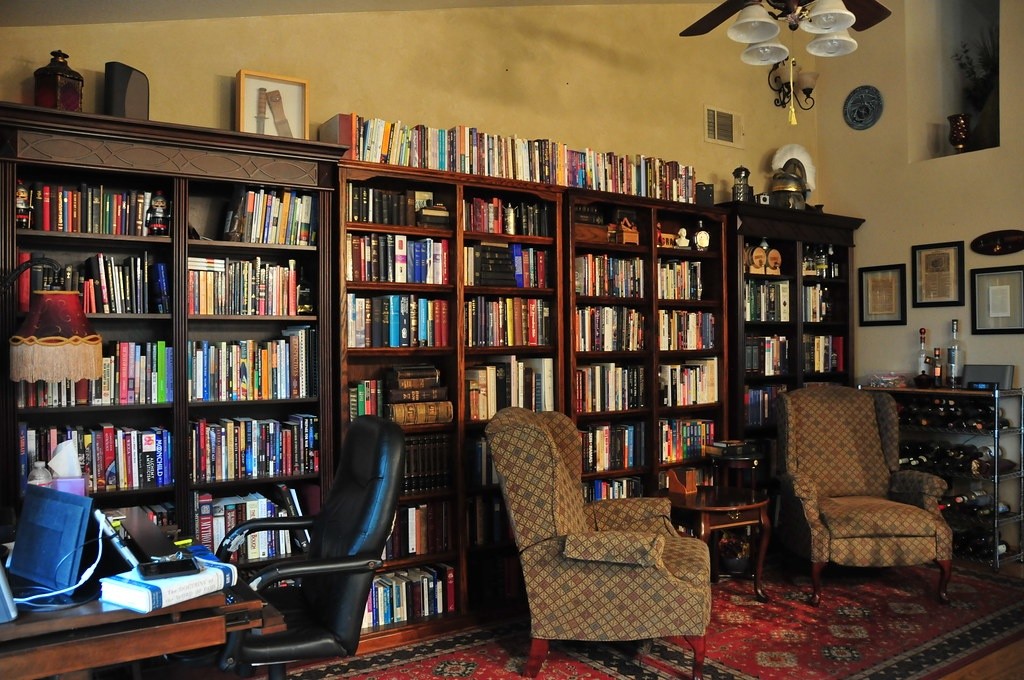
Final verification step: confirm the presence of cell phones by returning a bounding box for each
[135,557,201,581]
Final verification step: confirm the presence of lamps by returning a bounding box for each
[768,56,819,110]
[727,1,858,66]
[0,257,104,386]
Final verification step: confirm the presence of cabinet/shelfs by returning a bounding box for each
[857,384,1024,571]
[1,99,866,673]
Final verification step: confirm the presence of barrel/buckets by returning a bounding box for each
[764,249,782,268]
[743,247,766,268]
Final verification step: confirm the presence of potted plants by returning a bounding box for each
[952,22,1000,149]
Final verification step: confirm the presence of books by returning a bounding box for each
[318,114,719,632]
[742,247,844,428]
[15,178,321,615]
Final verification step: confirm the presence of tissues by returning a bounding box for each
[46,438,86,496]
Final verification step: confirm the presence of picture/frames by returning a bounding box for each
[912,240,964,308]
[857,263,907,326]
[235,69,311,141]
[970,265,1024,335]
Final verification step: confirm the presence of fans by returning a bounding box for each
[678,0,893,36]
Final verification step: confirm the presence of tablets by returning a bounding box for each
[92,508,146,568]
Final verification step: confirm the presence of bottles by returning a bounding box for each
[26,461,53,489]
[938,489,1011,519]
[896,398,1010,430]
[952,526,1010,559]
[916,328,930,376]
[898,440,1003,475]
[947,318,962,388]
[821,287,832,322]
[931,348,943,387]
[803,243,838,279]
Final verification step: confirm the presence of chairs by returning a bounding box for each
[775,384,952,606]
[485,406,711,680]
[210,413,408,679]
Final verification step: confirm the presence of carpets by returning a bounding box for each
[243,565,1024,680]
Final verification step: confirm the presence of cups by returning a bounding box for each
[913,370,931,389]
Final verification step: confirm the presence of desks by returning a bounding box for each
[1,544,287,680]
[652,485,770,603]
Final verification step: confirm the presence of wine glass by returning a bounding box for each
[946,114,971,154]
